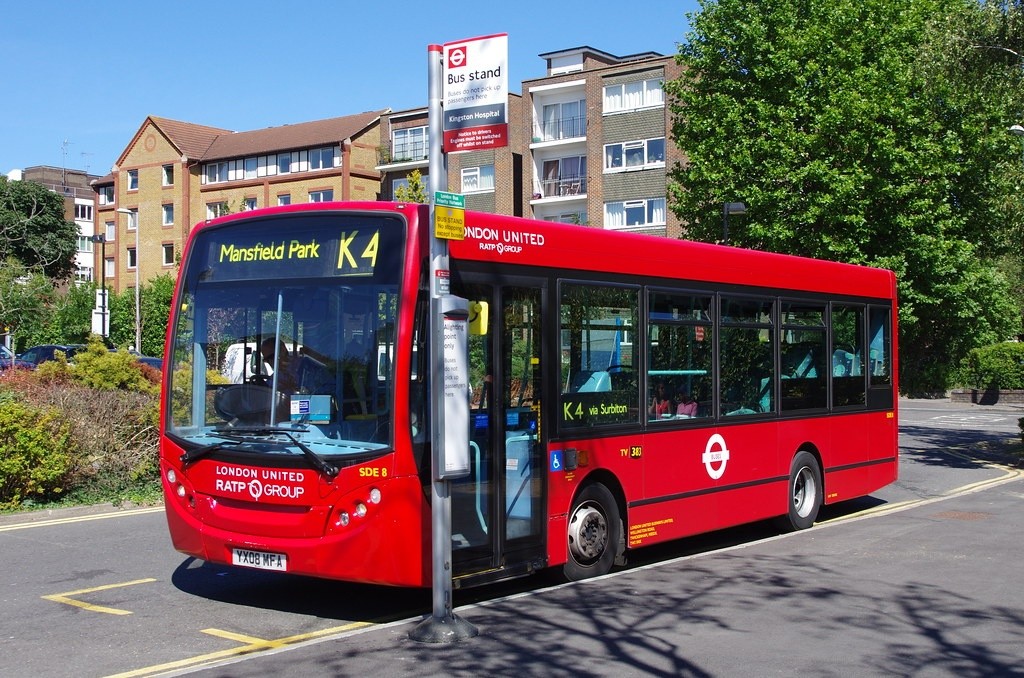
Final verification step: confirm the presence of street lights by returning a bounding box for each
[116,208,140,354]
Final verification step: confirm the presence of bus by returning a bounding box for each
[159,202,899,590]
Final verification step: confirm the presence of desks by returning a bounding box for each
[559,184,570,196]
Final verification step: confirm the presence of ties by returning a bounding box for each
[282,369,299,393]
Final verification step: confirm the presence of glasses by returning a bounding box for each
[262,349,282,363]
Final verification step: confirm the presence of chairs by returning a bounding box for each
[725,342,879,415]
[565,182,581,196]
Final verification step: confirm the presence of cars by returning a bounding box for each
[139,356,162,372]
[1,343,141,370]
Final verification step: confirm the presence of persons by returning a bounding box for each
[262,337,336,416]
[676,385,697,418]
[647,382,671,419]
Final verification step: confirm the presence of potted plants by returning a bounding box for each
[376,145,412,166]
[424,155,427,160]
[649,155,655,163]
